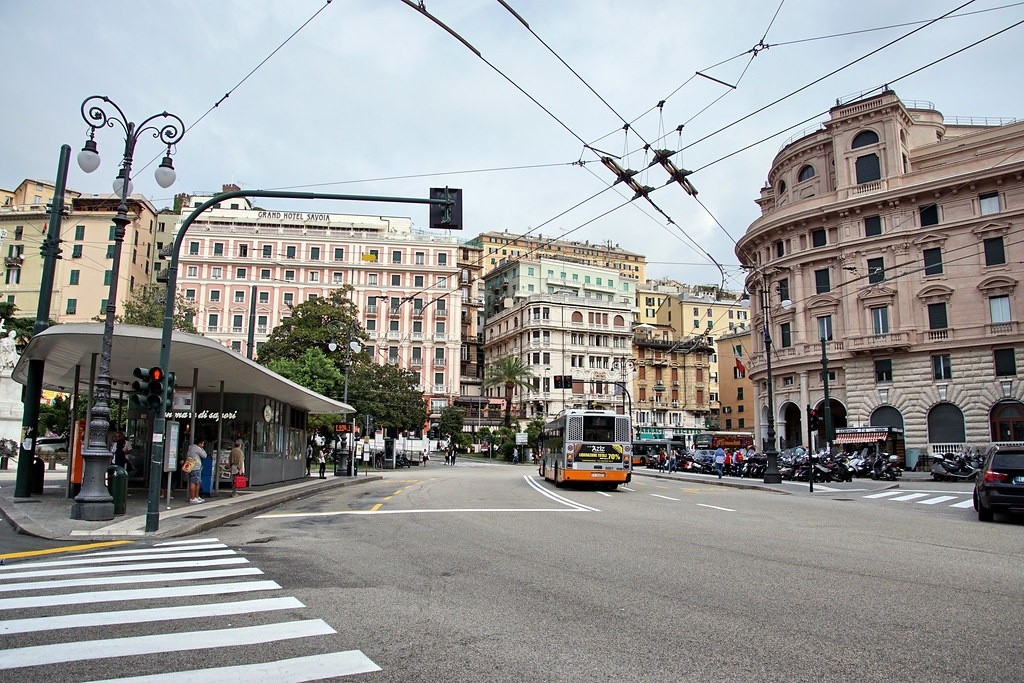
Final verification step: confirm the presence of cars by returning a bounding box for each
[35,437,68,452]
[972,444,1024,522]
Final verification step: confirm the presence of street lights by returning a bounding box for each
[71,95,185,522]
[327,321,361,476]
[763,282,792,483]
[545,368,550,422]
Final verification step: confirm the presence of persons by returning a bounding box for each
[746,445,756,456]
[375,450,386,466]
[659,448,667,473]
[228,438,244,498]
[512,447,518,465]
[724,448,733,476]
[444,444,458,466]
[422,449,428,467]
[186,440,208,504]
[109,430,134,473]
[437,439,441,452]
[668,449,679,473]
[304,443,340,479]
[396,450,411,468]
[713,444,727,480]
[732,447,744,479]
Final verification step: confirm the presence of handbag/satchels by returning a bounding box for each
[181,456,196,473]
[232,464,244,474]
[317,457,322,464]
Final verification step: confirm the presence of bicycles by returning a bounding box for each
[375,457,383,470]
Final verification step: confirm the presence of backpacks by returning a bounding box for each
[736,451,743,463]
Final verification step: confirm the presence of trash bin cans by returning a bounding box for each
[31,456,44,494]
[347,458,357,477]
[108,465,128,514]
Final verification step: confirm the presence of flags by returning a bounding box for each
[731,344,746,373]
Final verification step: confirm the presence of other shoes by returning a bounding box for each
[741,475,743,479]
[719,473,723,479]
[231,493,240,498]
[320,476,326,479]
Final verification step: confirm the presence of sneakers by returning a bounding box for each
[196,497,206,502]
[189,497,202,504]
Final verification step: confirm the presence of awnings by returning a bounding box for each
[831,426,904,445]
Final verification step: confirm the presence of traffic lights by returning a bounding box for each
[131,367,150,406]
[147,366,165,408]
[167,372,177,412]
[810,408,818,431]
[553,375,573,389]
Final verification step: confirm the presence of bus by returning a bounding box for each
[693,431,756,451]
[632,441,684,466]
[538,411,632,491]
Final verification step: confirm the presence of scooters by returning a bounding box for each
[396,453,410,469]
[930,452,987,484]
[647,447,903,483]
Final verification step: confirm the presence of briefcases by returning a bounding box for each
[234,472,247,489]
